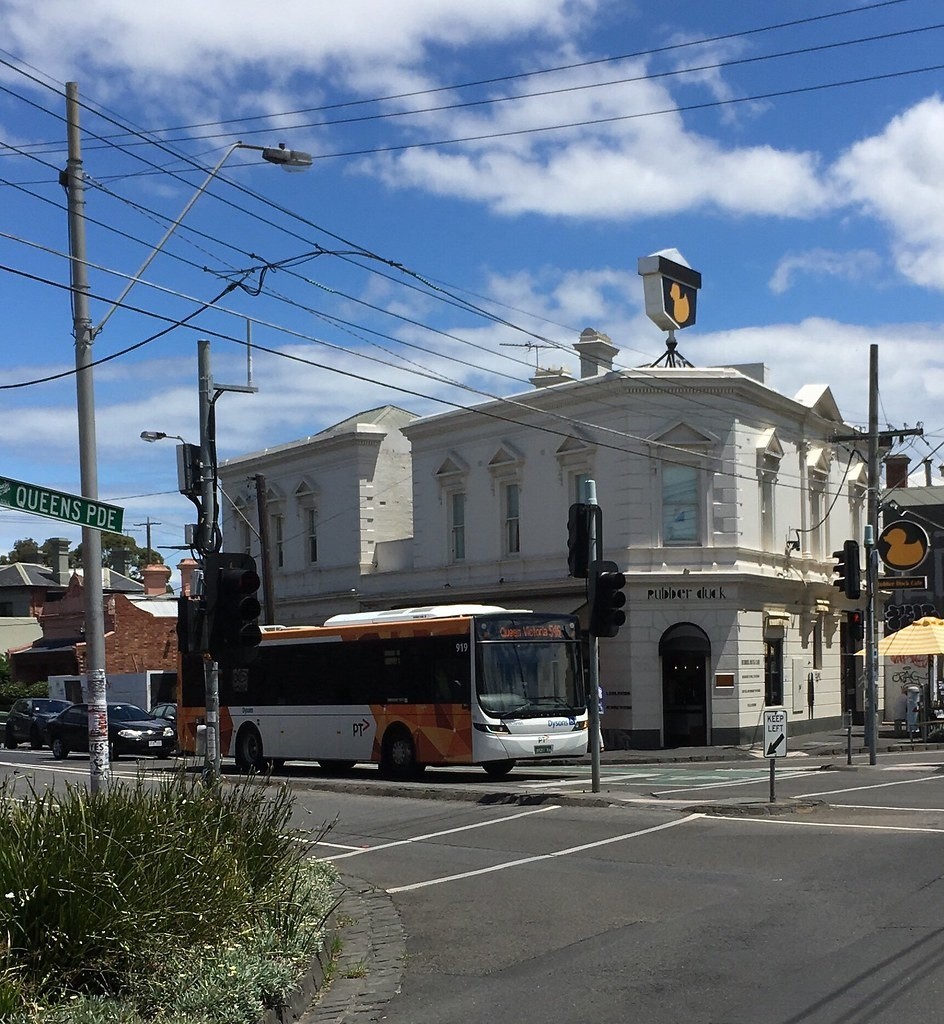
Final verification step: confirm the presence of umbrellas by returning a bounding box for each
[851,616,944,722]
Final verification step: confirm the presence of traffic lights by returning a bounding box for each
[832,540,860,599]
[841,608,865,641]
[589,560,627,638]
[203,552,262,663]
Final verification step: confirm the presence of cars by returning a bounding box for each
[4,698,75,751]
[147,702,178,751]
[47,701,177,759]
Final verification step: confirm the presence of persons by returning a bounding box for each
[892,687,908,722]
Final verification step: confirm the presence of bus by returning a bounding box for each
[176,603,590,776]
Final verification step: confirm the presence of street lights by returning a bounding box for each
[60,81,313,810]
[140,336,222,799]
